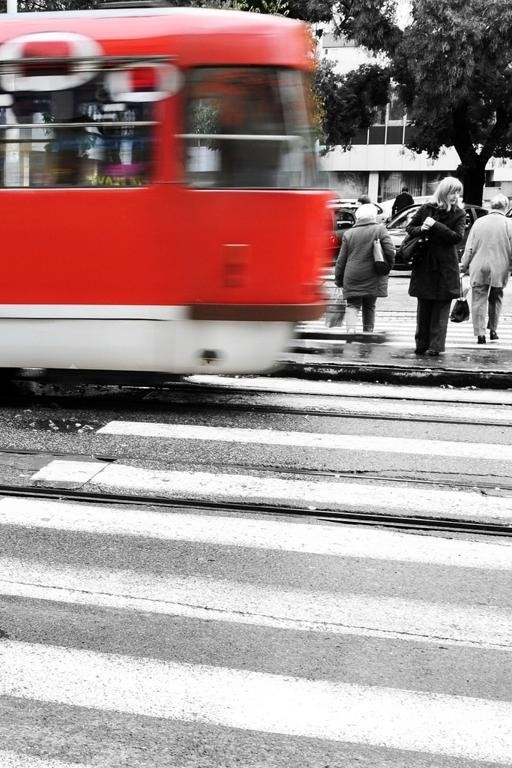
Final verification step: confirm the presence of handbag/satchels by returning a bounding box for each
[373,224,391,276]
[399,234,422,262]
[451,300,469,323]
[325,286,346,328]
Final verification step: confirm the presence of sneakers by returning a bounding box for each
[477,334,486,344]
[490,330,499,340]
[414,347,439,357]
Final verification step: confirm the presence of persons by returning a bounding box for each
[406,176,512,358]
[392,186,414,215]
[335,204,394,332]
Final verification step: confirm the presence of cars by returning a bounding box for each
[326,197,377,261]
[383,203,492,272]
[377,195,489,216]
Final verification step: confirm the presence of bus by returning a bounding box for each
[1,7,338,380]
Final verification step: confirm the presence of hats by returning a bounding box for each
[355,203,378,222]
